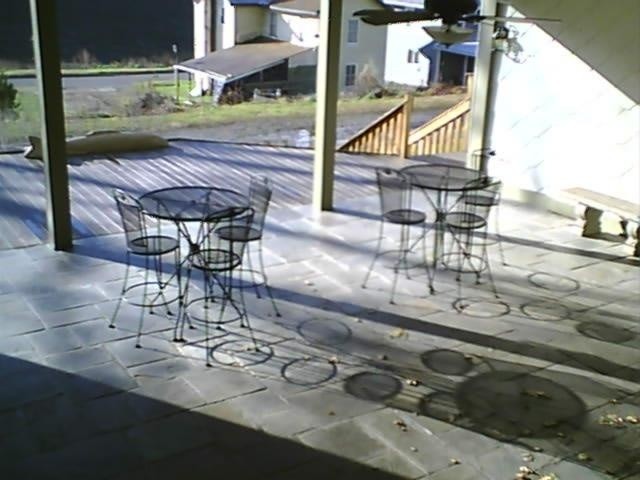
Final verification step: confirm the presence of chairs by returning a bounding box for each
[445,147,506,271]
[213,174,281,317]
[109,188,193,348]
[361,166,434,304]
[429,177,502,299]
[174,207,256,367]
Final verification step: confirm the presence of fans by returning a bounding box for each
[352,0,562,26]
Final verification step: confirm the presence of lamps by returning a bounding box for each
[492,27,510,51]
[422,24,474,49]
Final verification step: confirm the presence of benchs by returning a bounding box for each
[561,187,640,257]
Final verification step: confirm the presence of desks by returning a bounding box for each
[136,186,250,327]
[394,163,487,279]
